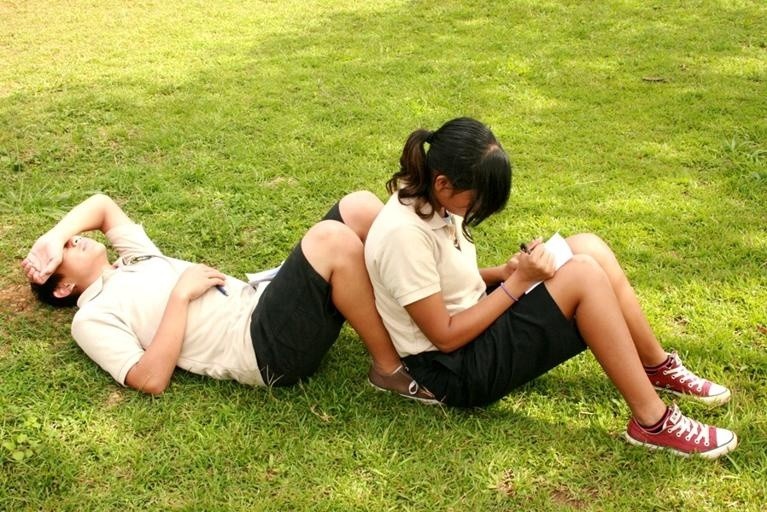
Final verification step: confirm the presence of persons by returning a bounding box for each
[362,114,740,460]
[20,187,446,405]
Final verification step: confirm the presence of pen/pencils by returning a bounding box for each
[520,243,531,255]
[215,285,228,296]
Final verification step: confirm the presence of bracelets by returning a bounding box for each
[499,281,520,304]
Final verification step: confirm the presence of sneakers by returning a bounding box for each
[626,406,738,459]
[645,351,732,405]
[367,364,442,404]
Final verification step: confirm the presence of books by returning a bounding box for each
[523,226,574,295]
